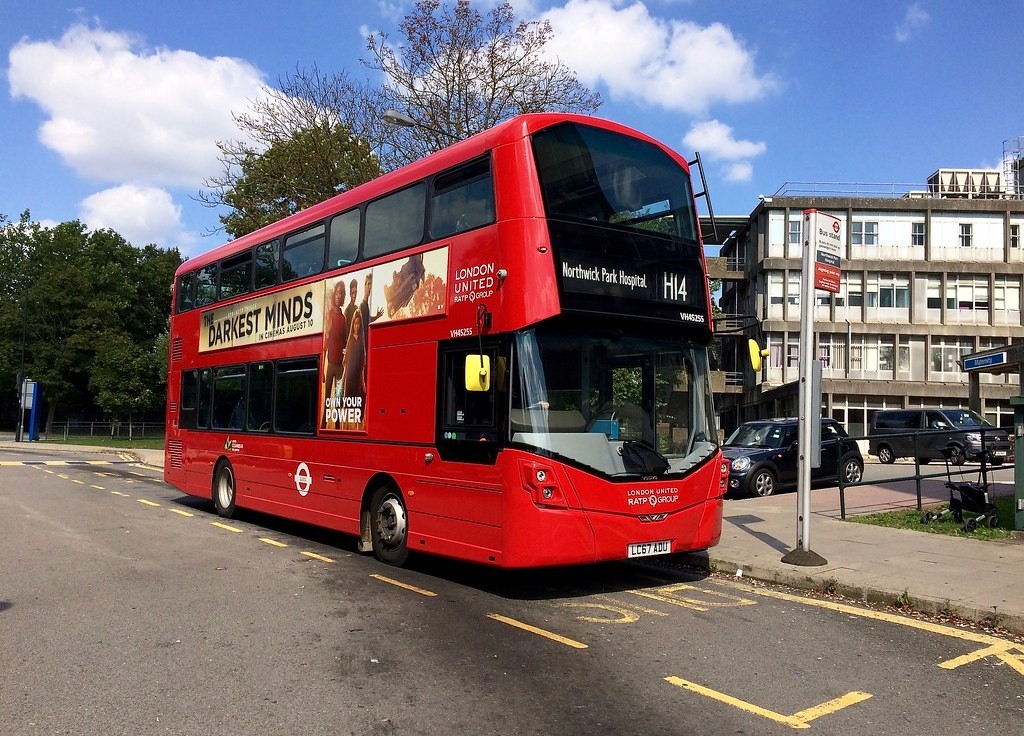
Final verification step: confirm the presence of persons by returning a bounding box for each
[512,387,550,409]
[321,274,384,429]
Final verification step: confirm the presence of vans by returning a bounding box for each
[866,408,1013,467]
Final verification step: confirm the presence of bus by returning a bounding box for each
[161,113,773,573]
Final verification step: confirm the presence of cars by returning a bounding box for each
[718,415,866,499]
